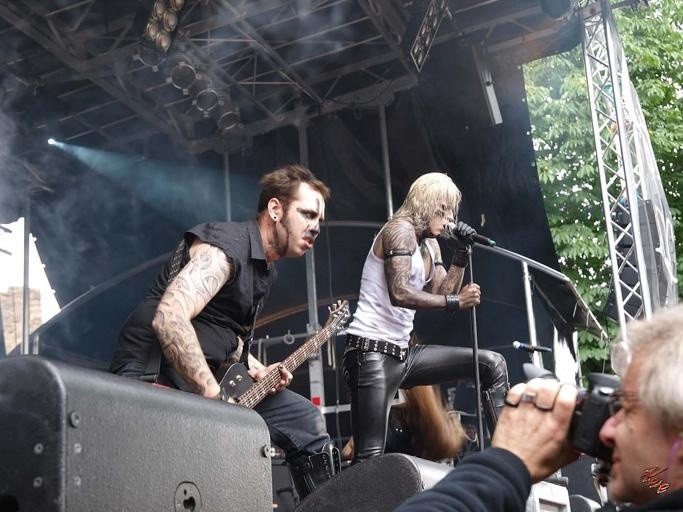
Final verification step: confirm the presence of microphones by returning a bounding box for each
[513,340,553,355]
[447,221,499,247]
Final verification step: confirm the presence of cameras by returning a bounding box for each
[517,361,620,468]
[568,462,570,465]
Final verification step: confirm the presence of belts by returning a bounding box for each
[343,335,409,363]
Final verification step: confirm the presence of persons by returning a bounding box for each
[335,172,511,464]
[385,385,466,465]
[394,303,683,511]
[111,164,341,507]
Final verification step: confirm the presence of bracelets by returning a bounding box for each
[446,293,461,314]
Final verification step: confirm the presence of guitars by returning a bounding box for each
[215,299,351,407]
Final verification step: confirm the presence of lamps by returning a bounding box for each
[400,0,448,78]
[119,0,244,141]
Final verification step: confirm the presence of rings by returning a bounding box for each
[522,391,537,403]
[503,399,519,408]
[534,403,554,412]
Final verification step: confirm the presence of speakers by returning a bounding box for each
[0,355,273,512]
